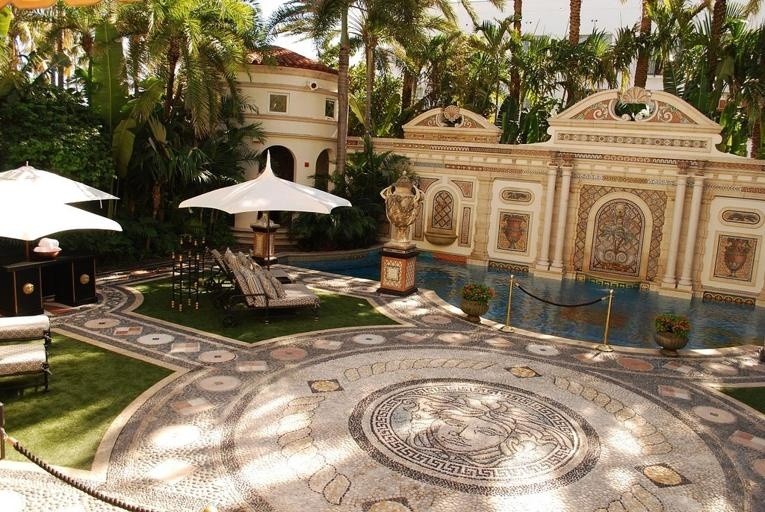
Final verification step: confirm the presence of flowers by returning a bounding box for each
[655,312,692,337]
[462,284,495,302]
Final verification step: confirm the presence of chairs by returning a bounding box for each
[208,248,320,327]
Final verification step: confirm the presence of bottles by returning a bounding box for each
[177,232,207,257]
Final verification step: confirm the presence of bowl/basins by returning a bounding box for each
[426,232,458,246]
[34,249,61,259]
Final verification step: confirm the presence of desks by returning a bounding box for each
[2,253,96,312]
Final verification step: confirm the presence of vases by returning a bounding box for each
[654,332,690,357]
[461,299,488,322]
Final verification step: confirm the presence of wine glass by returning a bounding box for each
[502,216,525,251]
[725,238,750,279]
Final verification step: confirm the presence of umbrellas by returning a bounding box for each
[0,204,124,259]
[0,161,121,205]
[178,149,353,272]
